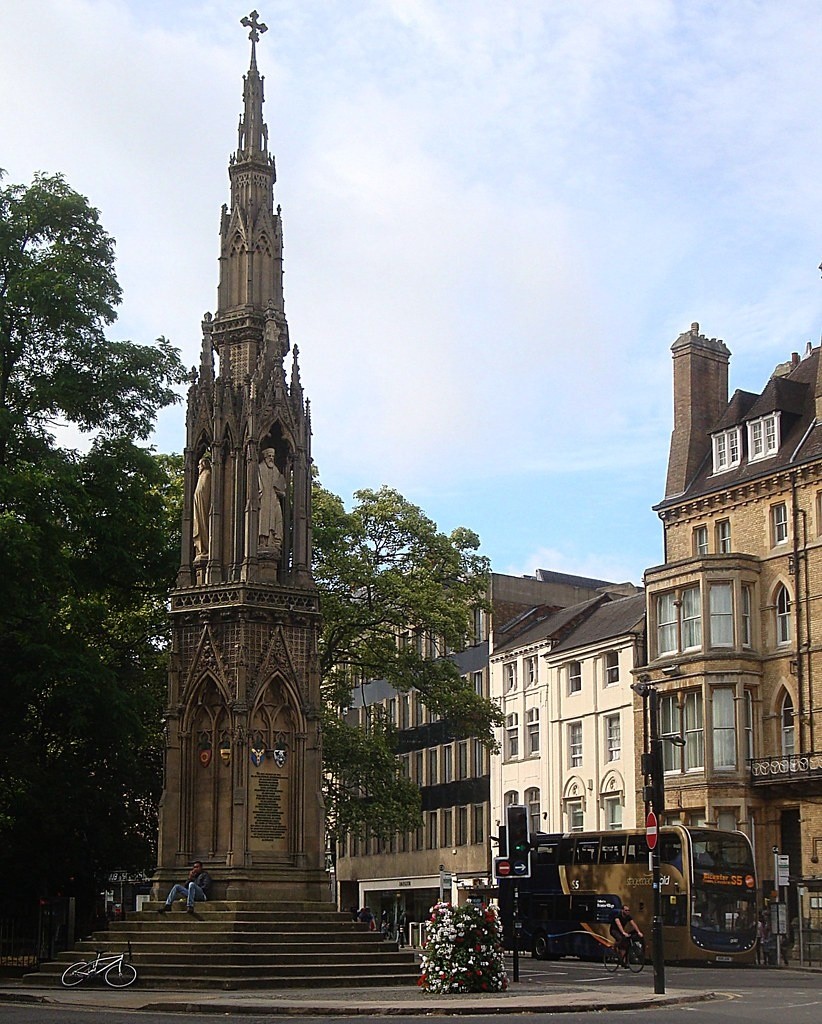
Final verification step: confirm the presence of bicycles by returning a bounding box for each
[602,934,646,973]
[61,940,138,988]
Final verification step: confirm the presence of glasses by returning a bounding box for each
[624,909,631,912]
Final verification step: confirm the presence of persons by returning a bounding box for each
[193,458,211,561]
[359,908,409,944]
[735,905,769,964]
[610,905,643,969]
[258,448,286,548]
[161,861,211,915]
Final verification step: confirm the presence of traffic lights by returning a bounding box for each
[507,807,532,857]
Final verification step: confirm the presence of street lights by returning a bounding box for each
[649,734,688,829]
[769,844,782,902]
[631,681,651,830]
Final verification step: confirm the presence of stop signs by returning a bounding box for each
[499,861,511,875]
[645,812,658,851]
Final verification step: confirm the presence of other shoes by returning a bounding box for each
[161,905,171,911]
[186,906,194,914]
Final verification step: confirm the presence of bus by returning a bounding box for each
[498,831,761,968]
